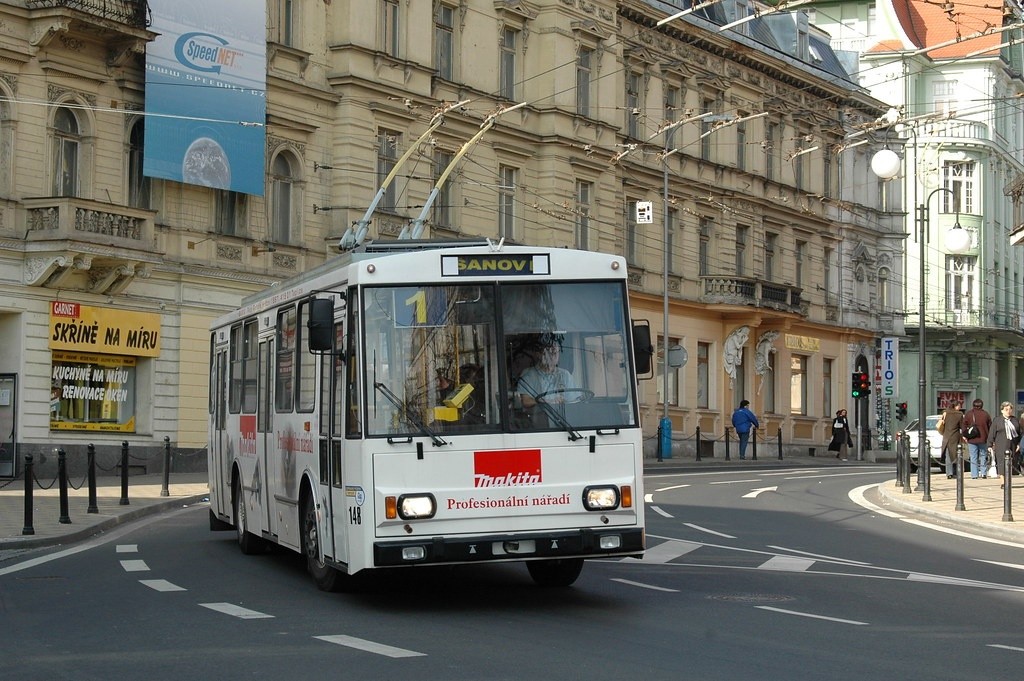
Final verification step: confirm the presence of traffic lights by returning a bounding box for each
[895,402,902,422]
[901,403,907,416]
[851,372,861,398]
[861,373,868,399]
[867,381,872,398]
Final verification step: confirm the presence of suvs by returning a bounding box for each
[895,414,972,472]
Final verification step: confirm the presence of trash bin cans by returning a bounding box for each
[701,440,715,457]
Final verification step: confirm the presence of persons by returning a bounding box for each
[723,327,750,389]
[987,401,1024,489]
[408,362,485,431]
[285,381,311,411]
[940,399,992,479]
[828,409,854,461]
[732,400,759,460]
[756,332,777,394]
[517,339,591,426]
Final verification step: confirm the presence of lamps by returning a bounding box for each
[252,243,277,257]
[187,233,218,250]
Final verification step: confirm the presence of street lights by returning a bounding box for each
[658,115,741,459]
[870,121,973,489]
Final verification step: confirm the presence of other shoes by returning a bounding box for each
[842,459,847,461]
[948,475,952,479]
[981,474,986,479]
[740,456,746,459]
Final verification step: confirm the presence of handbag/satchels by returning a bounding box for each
[834,419,843,428]
[936,412,946,435]
[989,448,997,478]
[965,425,979,439]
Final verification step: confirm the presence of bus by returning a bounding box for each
[205,98,644,596]
